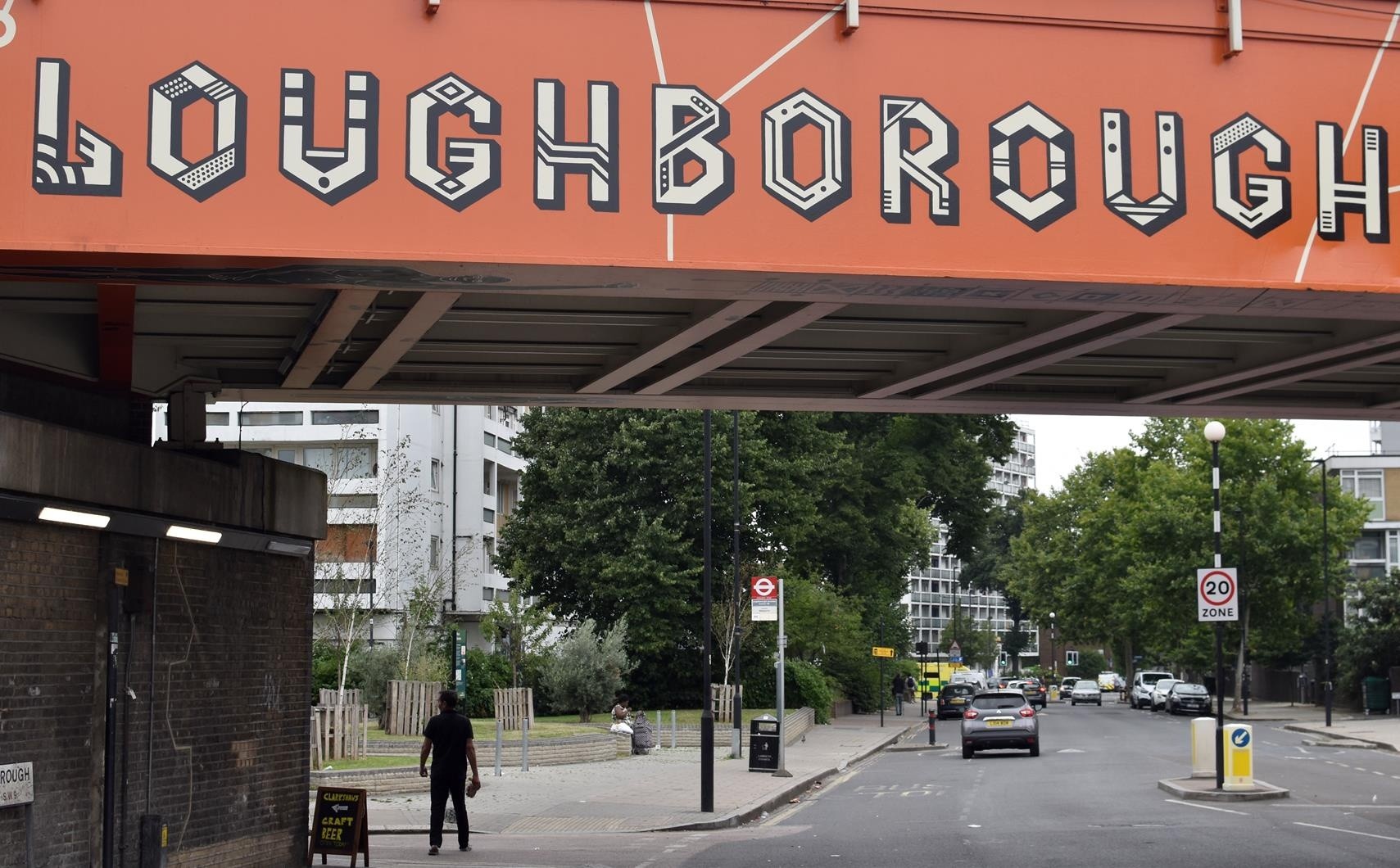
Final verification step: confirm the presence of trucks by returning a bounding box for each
[1098,672,1121,691]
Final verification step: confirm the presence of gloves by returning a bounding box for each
[420,761,428,777]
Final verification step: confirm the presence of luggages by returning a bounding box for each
[631,710,652,755]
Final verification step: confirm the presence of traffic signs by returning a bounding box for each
[312,788,360,857]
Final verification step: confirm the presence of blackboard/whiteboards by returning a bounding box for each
[312,785,368,855]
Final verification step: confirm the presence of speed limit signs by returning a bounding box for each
[1196,568,1238,622]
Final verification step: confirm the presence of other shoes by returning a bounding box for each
[428,846,438,856]
[461,845,470,851]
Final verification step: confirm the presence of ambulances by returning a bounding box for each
[913,661,971,701]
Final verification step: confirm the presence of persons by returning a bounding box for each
[892,674,904,716]
[611,694,636,738]
[420,690,482,855]
[905,673,918,704]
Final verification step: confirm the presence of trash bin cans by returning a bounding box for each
[749,713,780,773]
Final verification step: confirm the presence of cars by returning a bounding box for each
[935,671,1046,721]
[962,689,1042,759]
[1148,678,1212,717]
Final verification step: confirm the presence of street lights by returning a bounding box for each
[969,589,974,669]
[365,539,375,648]
[1048,611,1057,678]
[953,566,959,642]
[1303,459,1331,728]
[1204,422,1228,788]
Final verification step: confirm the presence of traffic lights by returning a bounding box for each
[999,651,1007,666]
[1066,651,1079,666]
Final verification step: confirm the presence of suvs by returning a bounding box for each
[1060,676,1102,706]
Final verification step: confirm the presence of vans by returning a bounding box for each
[1130,671,1174,707]
